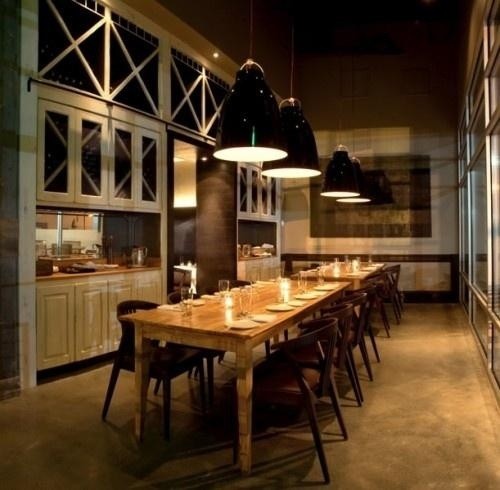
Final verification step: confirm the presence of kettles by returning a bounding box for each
[125,246,147,269]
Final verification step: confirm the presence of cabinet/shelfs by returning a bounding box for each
[236,162,282,281]
[22,0,170,375]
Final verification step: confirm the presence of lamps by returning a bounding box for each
[213,1,322,179]
[320,63,372,204]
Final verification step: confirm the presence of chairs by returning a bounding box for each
[100,263,402,482]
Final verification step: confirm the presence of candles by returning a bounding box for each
[333,258,341,275]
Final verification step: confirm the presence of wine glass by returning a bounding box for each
[178,255,383,332]
